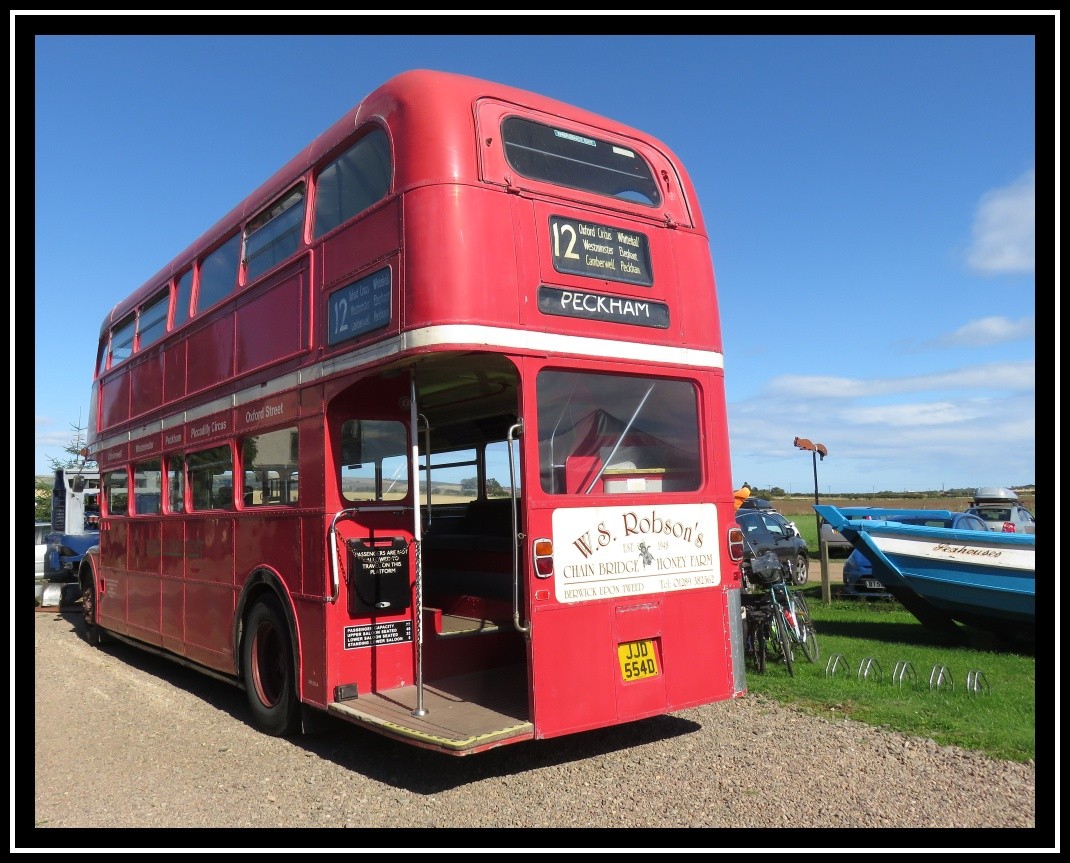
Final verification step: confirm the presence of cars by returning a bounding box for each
[818,514,872,558]
[842,546,894,601]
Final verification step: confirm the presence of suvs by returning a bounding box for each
[734,497,809,587]
[963,486,1035,536]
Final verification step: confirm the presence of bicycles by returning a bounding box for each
[740,549,819,678]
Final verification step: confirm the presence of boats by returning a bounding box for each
[811,505,1035,649]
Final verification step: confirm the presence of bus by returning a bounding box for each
[76,68,750,758]
